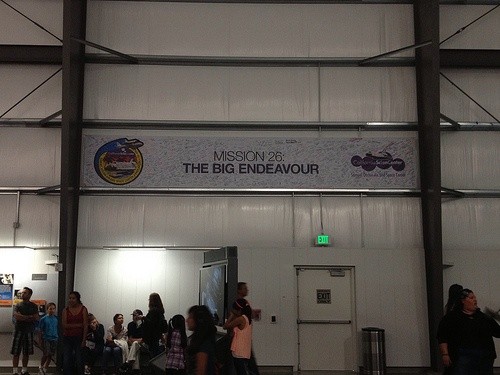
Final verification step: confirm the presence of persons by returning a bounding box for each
[61,292,89,375]
[444,283,463,315]
[231,281,259,375]
[134,292,166,375]
[439,288,500,375]
[88,305,224,375]
[223,297,253,375]
[10,285,39,375]
[36,302,58,375]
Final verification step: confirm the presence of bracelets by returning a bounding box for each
[441,354,449,356]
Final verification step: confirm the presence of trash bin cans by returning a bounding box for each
[361,326,386,375]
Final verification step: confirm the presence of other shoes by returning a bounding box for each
[39,367,45,375]
[21,371,30,375]
[13,373,18,375]
[43,368,49,375]
